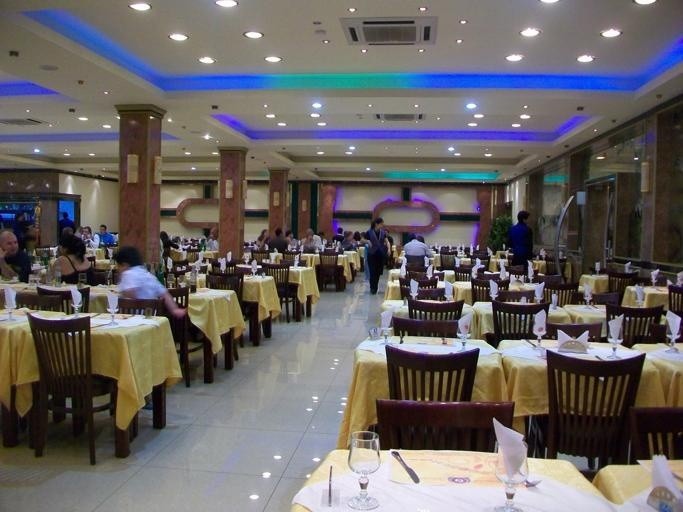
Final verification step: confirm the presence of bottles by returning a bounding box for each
[188,265,197,293]
[41,250,47,269]
[156,264,164,285]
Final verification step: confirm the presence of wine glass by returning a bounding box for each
[220,261,226,273]
[665,324,681,353]
[106,301,119,326]
[457,325,472,351]
[108,252,113,264]
[607,326,624,359]
[70,298,82,318]
[594,263,600,276]
[379,316,394,345]
[491,437,529,511]
[346,430,381,511]
[250,264,258,278]
[527,272,534,284]
[650,276,657,290]
[244,255,249,266]
[532,322,546,350]
[3,297,16,321]
[407,288,592,311]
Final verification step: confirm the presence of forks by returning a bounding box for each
[517,469,544,489]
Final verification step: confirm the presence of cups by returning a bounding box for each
[77,273,87,290]
[39,270,61,287]
[34,256,40,263]
[27,274,38,288]
[165,273,186,289]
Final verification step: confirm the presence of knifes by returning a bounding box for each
[89,324,107,329]
[591,305,598,309]
[390,448,421,483]
[524,338,536,347]
[90,313,99,318]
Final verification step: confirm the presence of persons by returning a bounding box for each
[510,211,534,266]
[1,212,219,317]
[257,217,433,295]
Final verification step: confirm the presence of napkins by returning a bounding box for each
[490,419,528,477]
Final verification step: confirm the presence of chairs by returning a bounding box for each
[164,286,204,386]
[374,398,517,453]
[626,406,683,463]
[0,236,681,347]
[383,346,481,400]
[24,311,135,466]
[525,348,647,471]
